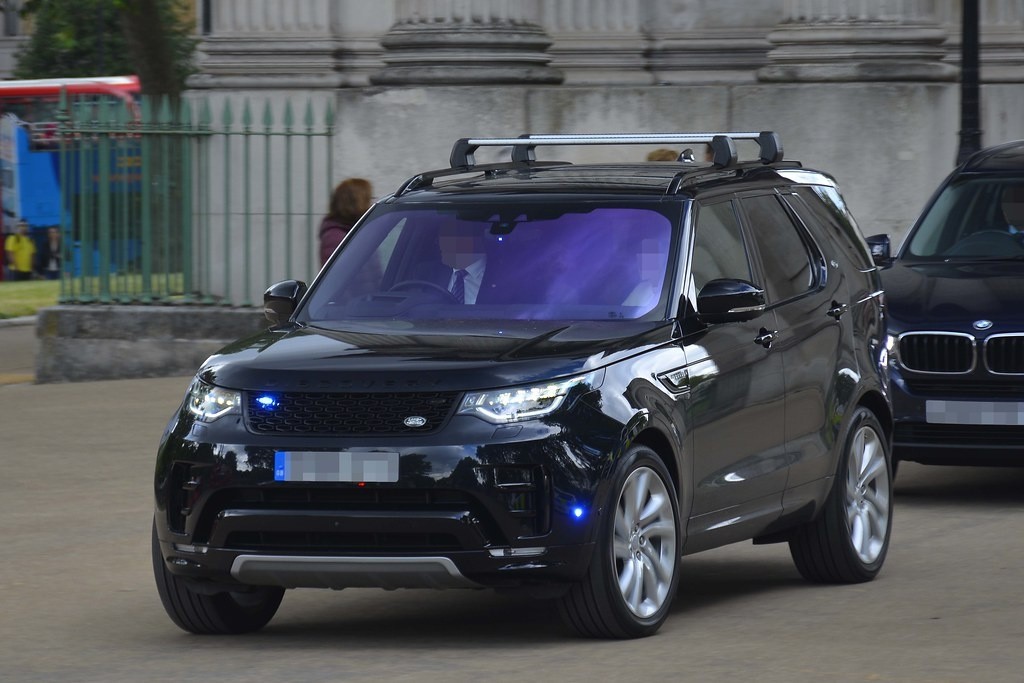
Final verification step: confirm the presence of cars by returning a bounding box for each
[865,140,1023,471]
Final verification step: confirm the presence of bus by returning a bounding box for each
[0,77,182,255]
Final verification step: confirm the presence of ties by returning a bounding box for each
[451,271,466,304]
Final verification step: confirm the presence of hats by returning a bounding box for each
[15,218,30,226]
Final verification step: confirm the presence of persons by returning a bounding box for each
[425,222,520,304]
[4,218,71,279]
[1001,184,1024,246]
[318,178,383,302]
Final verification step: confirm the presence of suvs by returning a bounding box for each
[151,131,897,636]
